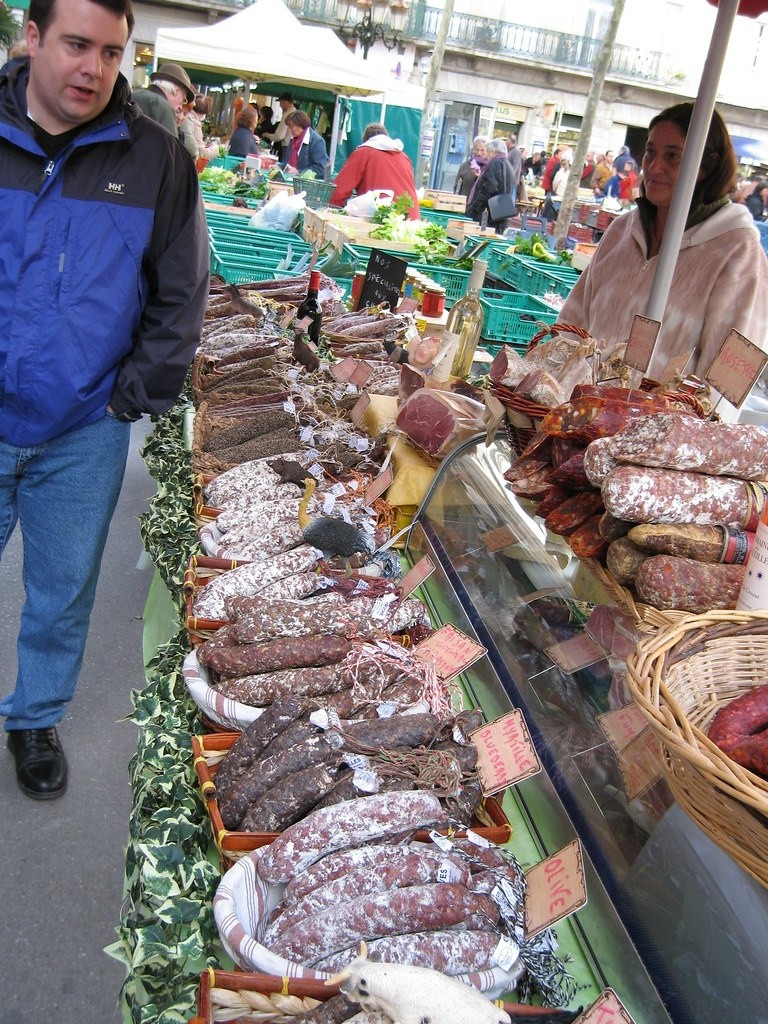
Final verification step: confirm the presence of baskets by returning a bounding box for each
[293,177,337,206]
[183,186,587,1024]
[490,322,704,466]
[626,609,768,889]
[561,529,697,638]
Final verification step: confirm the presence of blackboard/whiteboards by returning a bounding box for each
[357,247,408,312]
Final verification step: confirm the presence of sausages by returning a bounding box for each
[191,271,516,1024]
[503,384,768,613]
[708,685,768,781]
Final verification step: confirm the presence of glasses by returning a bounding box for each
[181,93,189,105]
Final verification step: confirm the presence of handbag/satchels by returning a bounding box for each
[248,190,307,232]
[344,188,394,218]
[488,194,518,220]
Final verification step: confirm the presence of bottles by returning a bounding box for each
[432,259,487,382]
[735,494,767,611]
[294,272,321,348]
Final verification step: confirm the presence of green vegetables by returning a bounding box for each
[197,147,268,200]
[367,191,475,270]
[516,231,574,268]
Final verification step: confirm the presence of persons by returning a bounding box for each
[130,62,196,139]
[551,102,768,411]
[262,93,296,162]
[190,94,219,160]
[0,0,210,801]
[730,173,768,222]
[229,106,262,156]
[453,135,638,235]
[176,100,197,157]
[330,124,421,220]
[255,106,273,143]
[275,110,328,180]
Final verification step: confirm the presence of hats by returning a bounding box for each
[150,63,195,103]
[275,92,293,101]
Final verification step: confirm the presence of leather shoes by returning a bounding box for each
[7,728,68,799]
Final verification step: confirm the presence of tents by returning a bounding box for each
[152,0,388,173]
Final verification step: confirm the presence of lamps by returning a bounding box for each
[222,82,232,90]
[232,78,245,89]
[250,81,258,89]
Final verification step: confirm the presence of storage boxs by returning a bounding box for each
[200,156,624,373]
